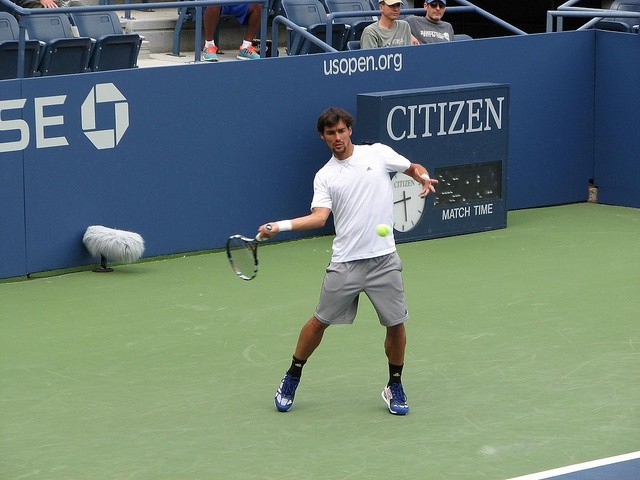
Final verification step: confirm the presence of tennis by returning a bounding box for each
[377,224,389,237]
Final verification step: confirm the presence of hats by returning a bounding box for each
[425,0,446,5]
[378,0,405,6]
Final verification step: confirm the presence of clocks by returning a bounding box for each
[390,172,427,232]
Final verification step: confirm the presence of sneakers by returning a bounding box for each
[382,382,408,415]
[202,44,219,62]
[274,372,300,412]
[237,45,260,60]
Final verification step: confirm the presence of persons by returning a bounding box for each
[402,0,455,45]
[9,0,85,9]
[257,105,440,416]
[359,0,412,50]
[201,4,262,62]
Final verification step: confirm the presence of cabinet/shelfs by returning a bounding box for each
[357,82,512,244]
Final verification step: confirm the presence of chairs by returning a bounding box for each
[71,12,143,70]
[454,34,472,41]
[371,0,414,20]
[325,1,378,41]
[592,1,640,32]
[170,1,239,56]
[281,1,346,53]
[29,15,94,75]
[346,41,362,50]
[0,12,40,78]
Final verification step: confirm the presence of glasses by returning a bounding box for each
[426,2,446,9]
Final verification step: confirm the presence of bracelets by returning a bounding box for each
[421,173,430,180]
[276,220,293,232]
